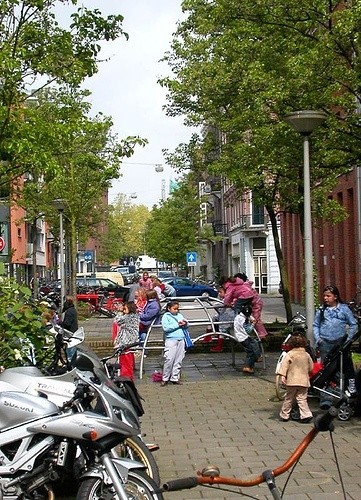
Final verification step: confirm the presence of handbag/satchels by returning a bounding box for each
[182,328,194,348]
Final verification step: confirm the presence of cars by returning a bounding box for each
[77,265,141,285]
[51,278,130,303]
[157,271,218,298]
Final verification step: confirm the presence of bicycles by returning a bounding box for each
[151,395,347,500]
[275,312,307,400]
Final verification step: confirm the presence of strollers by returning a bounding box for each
[289,330,361,420]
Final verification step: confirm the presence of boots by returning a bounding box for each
[201,329,213,343]
[210,337,225,352]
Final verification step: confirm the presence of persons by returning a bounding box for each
[278,332,314,422]
[134,287,159,354]
[139,272,176,301]
[161,301,189,386]
[114,301,140,350]
[61,295,78,362]
[312,286,358,386]
[212,273,268,342]
[233,306,261,373]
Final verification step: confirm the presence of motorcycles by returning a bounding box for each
[0,326,164,500]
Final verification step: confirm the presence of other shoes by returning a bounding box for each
[172,381,181,384]
[243,366,254,373]
[300,417,313,423]
[283,418,289,422]
[259,332,268,337]
[160,381,168,386]
